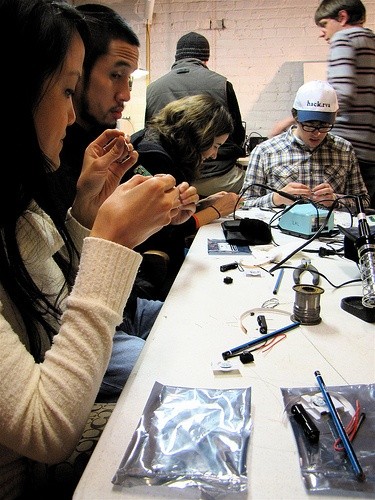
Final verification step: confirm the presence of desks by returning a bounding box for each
[71,207,375,500]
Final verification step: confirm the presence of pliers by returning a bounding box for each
[293,256,319,285]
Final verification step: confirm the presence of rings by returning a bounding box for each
[116,158,123,163]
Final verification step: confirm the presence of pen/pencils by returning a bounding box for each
[314,368,366,482]
[272,268,284,294]
[221,322,301,360]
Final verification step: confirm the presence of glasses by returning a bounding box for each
[300,122,334,133]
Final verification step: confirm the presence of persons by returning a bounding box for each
[271,0,375,209]
[0,0,246,500]
[240,80,370,212]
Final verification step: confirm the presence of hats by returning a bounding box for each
[292,80,339,123]
[175,32,210,61]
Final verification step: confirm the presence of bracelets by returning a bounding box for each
[209,205,221,220]
